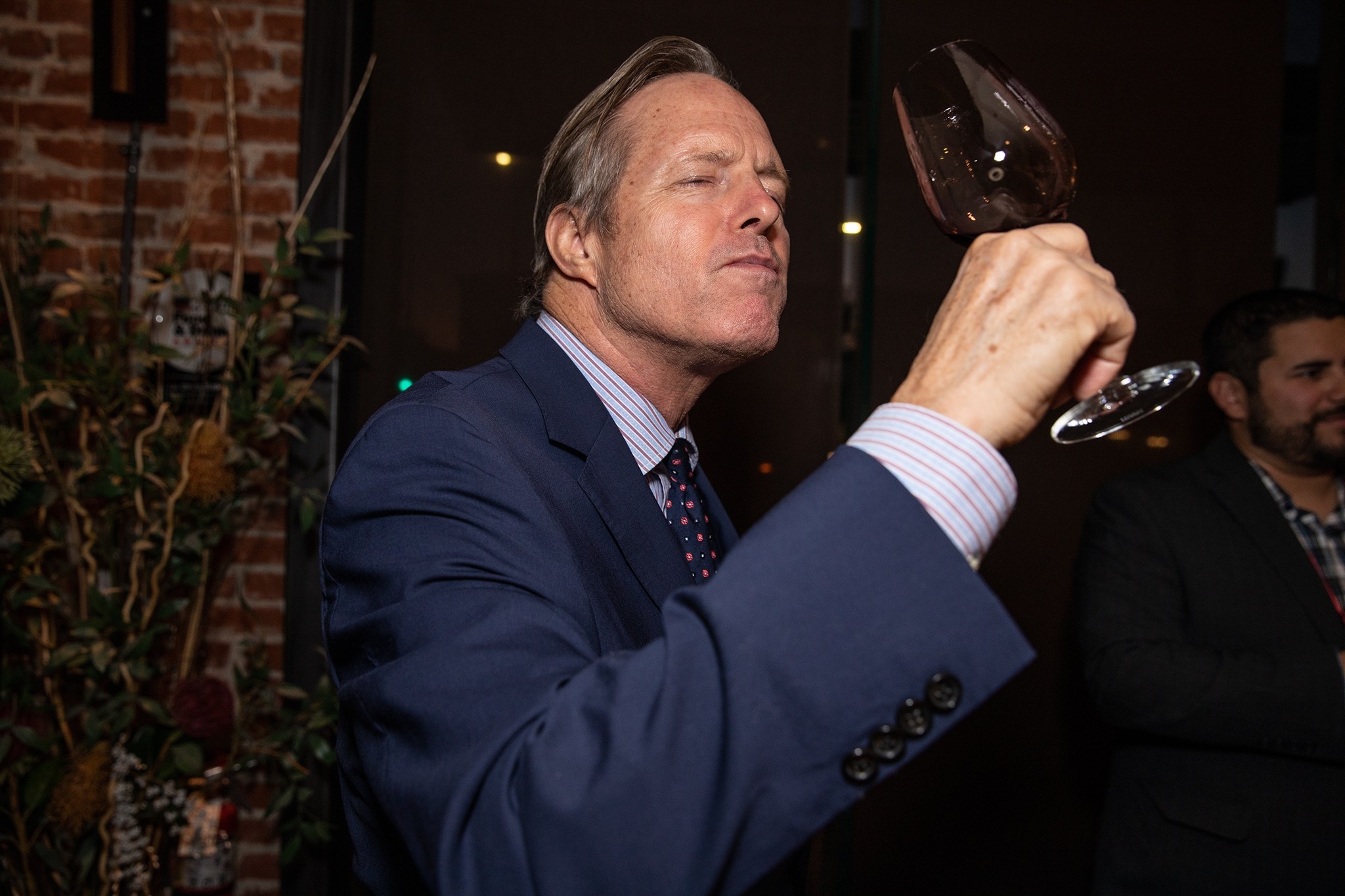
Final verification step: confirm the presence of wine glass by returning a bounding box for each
[890,35,1204,450]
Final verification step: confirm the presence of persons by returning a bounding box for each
[1091,283,1345,896]
[306,32,1137,893]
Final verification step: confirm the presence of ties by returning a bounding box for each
[662,440,721,586]
